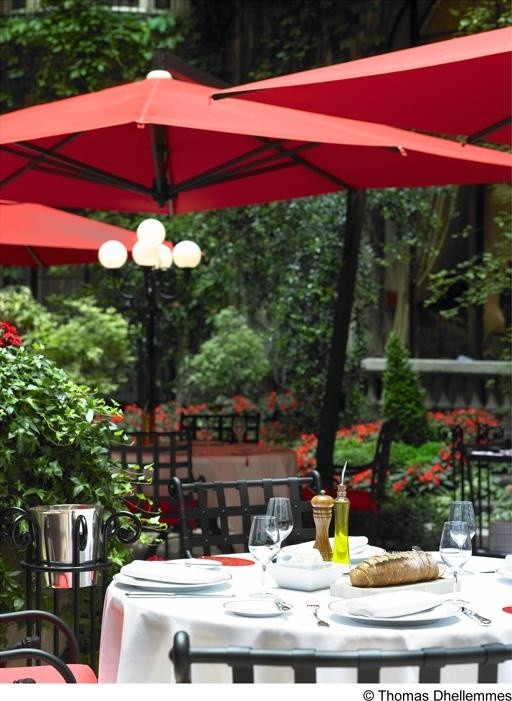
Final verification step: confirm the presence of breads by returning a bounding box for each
[350,551,439,587]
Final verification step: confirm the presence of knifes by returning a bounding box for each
[459,607,491,625]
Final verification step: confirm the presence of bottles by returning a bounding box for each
[332,485,353,570]
[309,490,335,561]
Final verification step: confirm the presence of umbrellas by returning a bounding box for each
[0,68,509,216]
[206,24,512,147]
[0,198,205,267]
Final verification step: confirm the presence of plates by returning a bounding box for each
[495,566,512,585]
[328,593,459,628]
[284,544,386,564]
[223,597,293,618]
[113,559,233,595]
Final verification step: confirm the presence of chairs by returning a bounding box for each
[107,426,209,530]
[300,415,399,548]
[0,608,99,683]
[167,470,322,558]
[165,631,511,682]
[178,409,262,445]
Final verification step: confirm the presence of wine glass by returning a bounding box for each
[265,498,293,574]
[248,516,281,599]
[439,522,474,606]
[447,501,476,577]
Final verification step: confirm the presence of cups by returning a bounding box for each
[29,503,103,590]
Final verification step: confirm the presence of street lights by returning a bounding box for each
[98,217,202,442]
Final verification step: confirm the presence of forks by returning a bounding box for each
[307,604,329,628]
